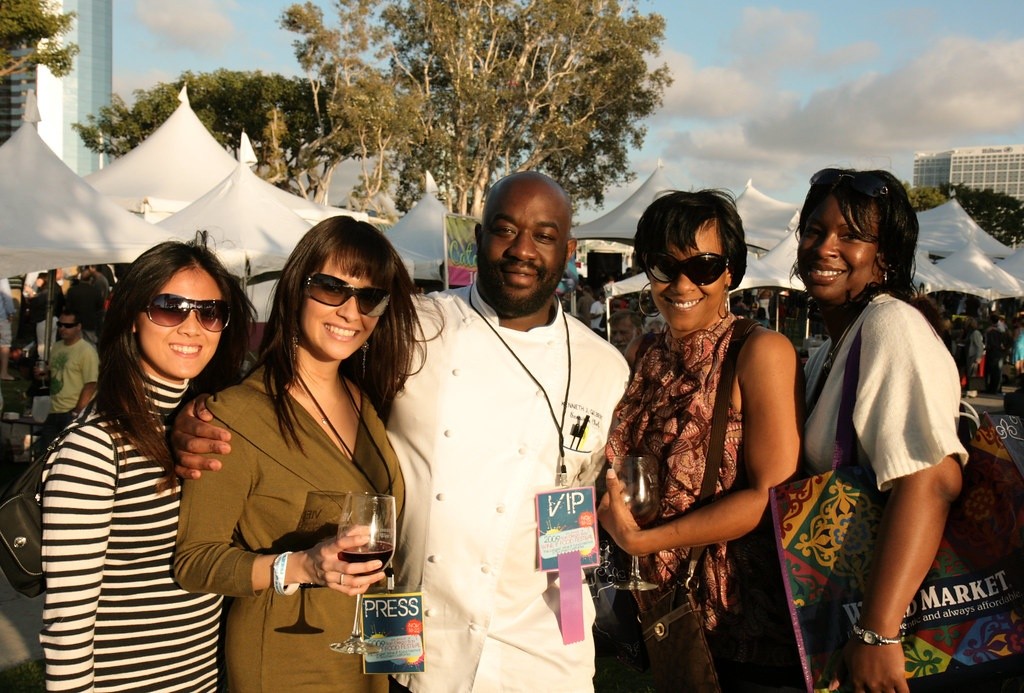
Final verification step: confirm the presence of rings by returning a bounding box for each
[339,573,345,586]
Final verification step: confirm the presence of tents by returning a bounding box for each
[0,78,1024,323]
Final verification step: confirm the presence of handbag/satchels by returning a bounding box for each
[0,415,119,598]
[638,586,720,693]
[769,300,1024,693]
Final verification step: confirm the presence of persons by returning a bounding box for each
[175,172,630,693]
[172,216,406,693]
[595,187,799,693]
[771,169,971,693]
[1,249,1024,457]
[41,241,248,693]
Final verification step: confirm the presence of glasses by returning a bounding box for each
[641,248,730,285]
[810,169,890,219]
[300,274,390,316]
[137,293,234,332]
[55,321,79,329]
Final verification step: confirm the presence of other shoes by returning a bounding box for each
[1,376,21,381]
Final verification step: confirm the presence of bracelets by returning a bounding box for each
[272,551,300,596]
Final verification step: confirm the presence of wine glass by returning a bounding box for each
[36,360,49,389]
[327,492,396,656]
[609,456,660,592]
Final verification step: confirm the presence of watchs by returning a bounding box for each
[852,623,904,645]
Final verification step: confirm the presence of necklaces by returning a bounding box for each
[823,306,863,370]
[297,385,338,425]
